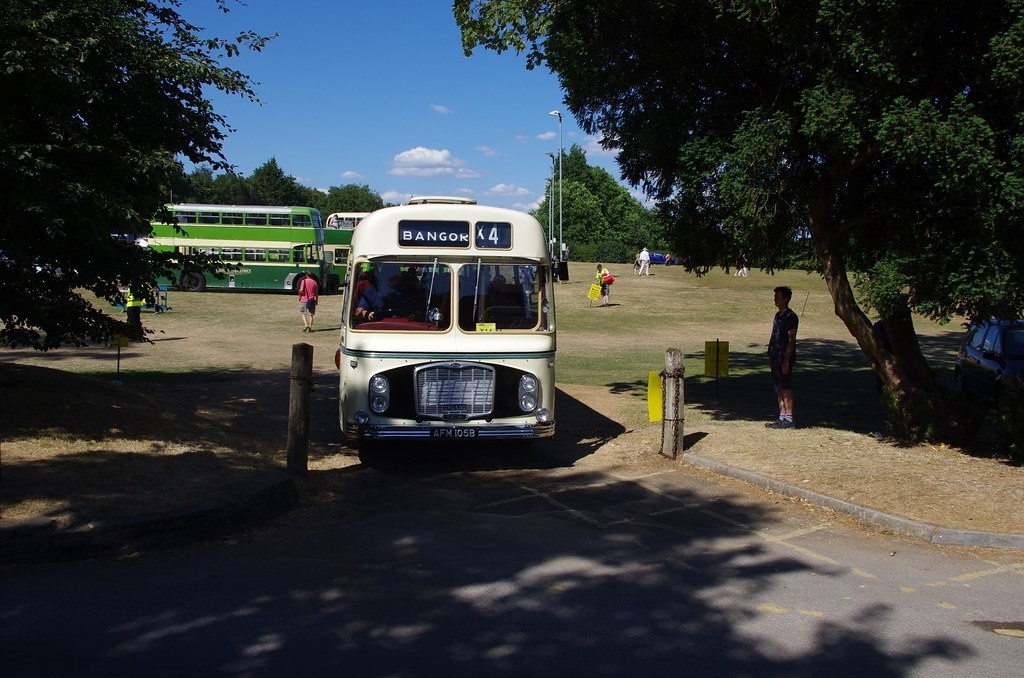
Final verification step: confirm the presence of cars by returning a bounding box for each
[954,319,1024,434]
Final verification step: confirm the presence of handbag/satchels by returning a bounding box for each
[602,273,614,285]
[306,299,316,310]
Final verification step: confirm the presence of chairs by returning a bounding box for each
[353,271,370,305]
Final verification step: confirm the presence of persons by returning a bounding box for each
[552,253,559,279]
[665,254,671,266]
[563,245,570,261]
[764,286,800,430]
[633,247,651,276]
[299,270,318,333]
[596,264,611,308]
[329,214,338,229]
[355,263,525,321]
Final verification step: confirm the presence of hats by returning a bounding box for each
[642,247,647,251]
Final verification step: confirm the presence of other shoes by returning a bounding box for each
[303,326,312,332]
[765,418,794,428]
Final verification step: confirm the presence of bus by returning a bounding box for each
[127,203,371,293]
[339,196,556,448]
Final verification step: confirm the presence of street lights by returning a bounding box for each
[545,110,565,264]
